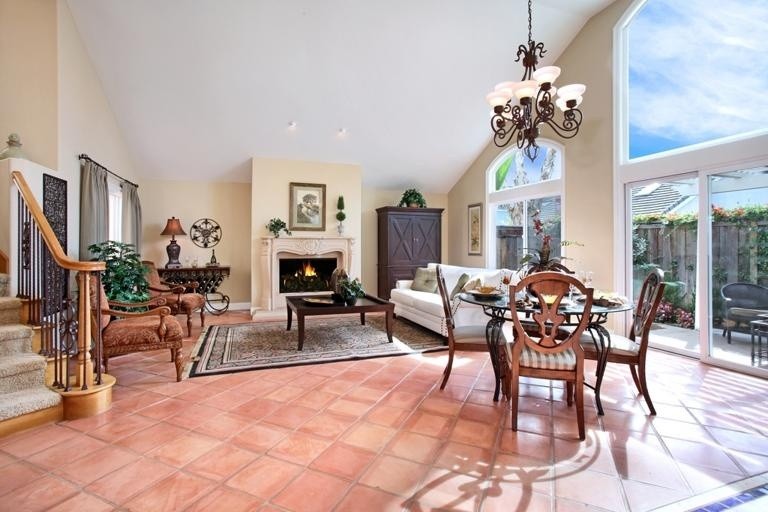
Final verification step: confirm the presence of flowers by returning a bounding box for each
[513,209,585,276]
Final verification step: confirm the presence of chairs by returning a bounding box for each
[435,264,509,400]
[75,269,186,383]
[719,282,767,343]
[574,267,666,415]
[512,264,571,344]
[141,260,206,337]
[508,271,594,441]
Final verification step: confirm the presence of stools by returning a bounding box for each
[749,319,767,364]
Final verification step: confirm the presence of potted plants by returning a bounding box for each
[265,218,291,239]
[336,278,366,306]
[397,188,428,209]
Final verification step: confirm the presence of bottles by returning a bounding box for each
[210,249,217,264]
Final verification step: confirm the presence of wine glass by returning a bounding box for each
[565,272,597,305]
[184,256,198,268]
[500,271,512,300]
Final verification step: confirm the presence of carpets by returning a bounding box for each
[181,308,451,379]
[336,194,347,235]
[636,465,767,512]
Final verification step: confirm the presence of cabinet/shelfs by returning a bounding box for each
[375,206,445,266]
[376,266,426,301]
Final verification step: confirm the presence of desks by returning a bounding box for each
[458,286,636,417]
[285,291,396,351]
[157,265,233,316]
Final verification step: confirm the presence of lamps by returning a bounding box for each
[486,1,588,162]
[160,216,188,268]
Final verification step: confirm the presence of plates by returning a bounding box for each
[531,295,576,309]
[466,290,504,298]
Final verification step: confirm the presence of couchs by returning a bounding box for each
[388,262,527,345]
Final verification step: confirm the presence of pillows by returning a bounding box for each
[460,276,481,293]
[410,266,442,294]
[449,273,470,300]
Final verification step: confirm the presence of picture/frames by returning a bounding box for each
[467,202,483,256]
[288,181,327,232]
